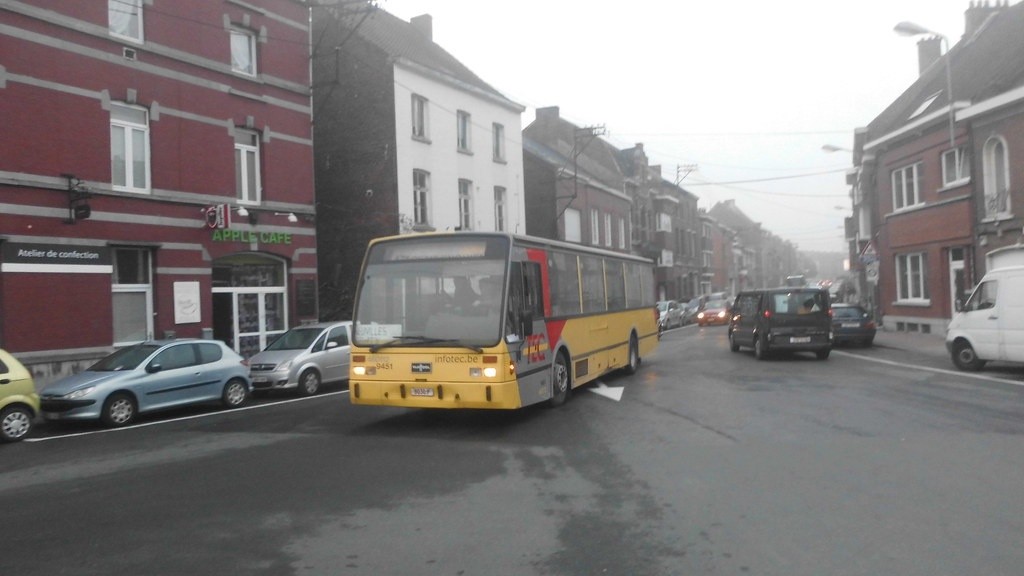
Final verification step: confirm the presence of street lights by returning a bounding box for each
[822,145,881,326]
[893,20,956,149]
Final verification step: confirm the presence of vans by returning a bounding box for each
[728,287,830,361]
[945,265,1024,370]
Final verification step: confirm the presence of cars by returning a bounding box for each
[679,295,704,323]
[40,340,254,427]
[658,300,683,330]
[809,268,845,304]
[0,348,40,442]
[830,303,878,348]
[704,293,728,303]
[248,320,353,400]
[698,300,731,326]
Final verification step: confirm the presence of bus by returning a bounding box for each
[349,231,658,408]
[787,274,806,287]
